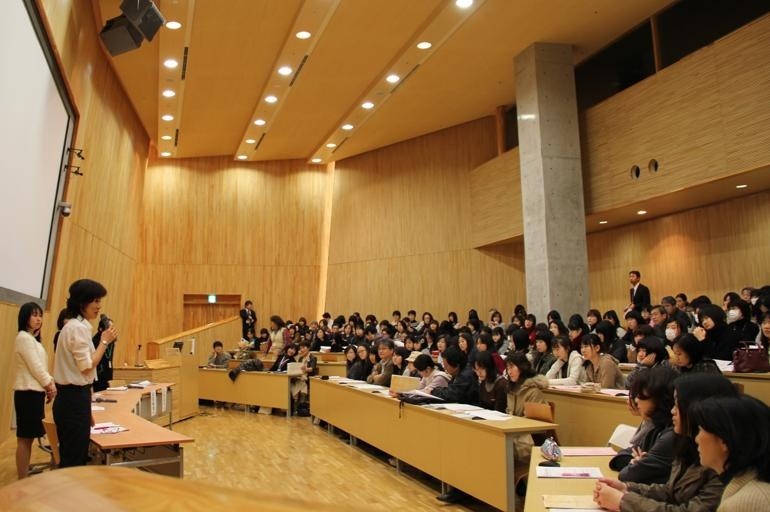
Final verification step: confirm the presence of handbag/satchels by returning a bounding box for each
[524,399,559,445]
[243,358,264,371]
[733,348,770,373]
[540,438,561,461]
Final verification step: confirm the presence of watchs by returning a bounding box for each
[97,338,111,351]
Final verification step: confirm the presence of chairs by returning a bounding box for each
[28,418,62,476]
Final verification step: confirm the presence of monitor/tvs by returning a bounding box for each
[173,341,184,352]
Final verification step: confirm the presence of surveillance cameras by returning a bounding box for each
[58,202,72,217]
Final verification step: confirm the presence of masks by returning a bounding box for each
[728,310,742,323]
[665,328,677,342]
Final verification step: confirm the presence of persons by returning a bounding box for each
[208,306,628,416]
[14,300,56,479]
[240,301,257,340]
[54,309,72,352]
[623,271,650,318]
[621,286,770,361]
[53,279,119,466]
[89,313,118,397]
[592,334,770,512]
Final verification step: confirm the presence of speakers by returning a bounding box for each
[99,13,145,58]
[119,0,165,43]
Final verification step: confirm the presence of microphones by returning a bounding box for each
[127,385,144,389]
[100,314,109,329]
[96,398,117,402]
[135,344,144,367]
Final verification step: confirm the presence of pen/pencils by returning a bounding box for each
[94,426,112,430]
[563,472,591,478]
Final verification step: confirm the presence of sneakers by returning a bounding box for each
[389,458,396,466]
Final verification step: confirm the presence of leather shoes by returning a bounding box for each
[436,490,465,501]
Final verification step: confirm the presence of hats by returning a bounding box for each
[405,351,423,362]
